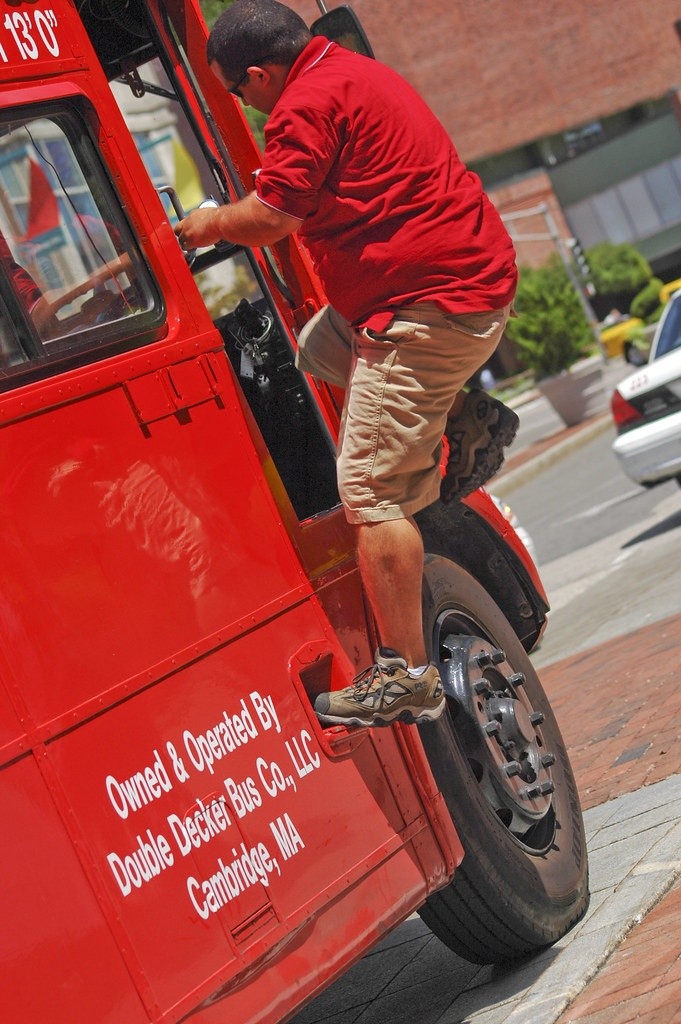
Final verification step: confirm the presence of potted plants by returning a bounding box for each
[500,251,610,427]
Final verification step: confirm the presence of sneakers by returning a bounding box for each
[312,647,446,727]
[441,388,522,507]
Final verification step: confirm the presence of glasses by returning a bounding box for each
[229,53,278,97]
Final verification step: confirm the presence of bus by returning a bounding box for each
[3,0,594,1024]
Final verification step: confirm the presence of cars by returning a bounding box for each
[610,283,681,490]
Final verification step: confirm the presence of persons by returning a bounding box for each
[171,0,519,728]
[0,215,128,341]
